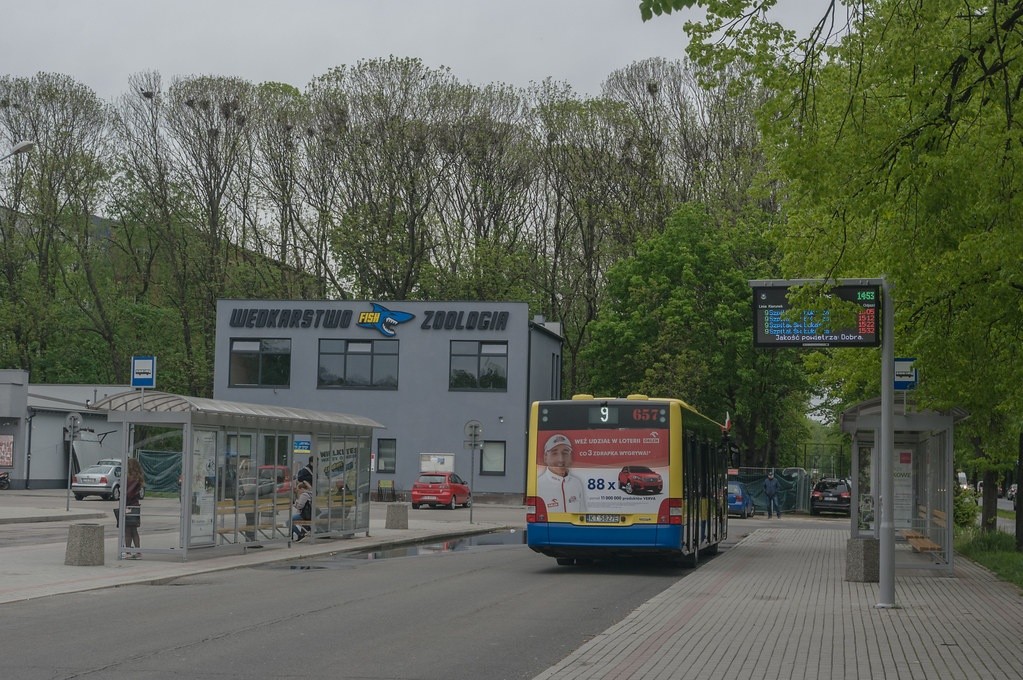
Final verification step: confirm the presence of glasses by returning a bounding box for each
[241,467,248,470]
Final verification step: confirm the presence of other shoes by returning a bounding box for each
[247,545,263,548]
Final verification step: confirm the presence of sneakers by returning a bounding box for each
[126,553,143,560]
[121,552,130,558]
[301,525,311,534]
[295,534,305,542]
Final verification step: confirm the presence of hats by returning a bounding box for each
[543,434,572,455]
[768,472,773,475]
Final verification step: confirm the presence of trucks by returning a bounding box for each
[958,472,968,490]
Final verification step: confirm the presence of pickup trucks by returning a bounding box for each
[976,480,983,494]
[252,465,297,493]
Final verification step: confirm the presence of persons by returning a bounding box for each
[113,457,142,560]
[537,434,586,513]
[762,472,781,519]
[293,456,319,541]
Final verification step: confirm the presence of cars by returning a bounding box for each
[810,477,851,517]
[998,487,1005,498]
[71,459,146,501]
[782,467,808,478]
[728,481,756,519]
[411,471,472,511]
[1007,484,1017,510]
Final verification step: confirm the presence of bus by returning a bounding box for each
[521,393,741,569]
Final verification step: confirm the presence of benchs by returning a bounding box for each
[216,518,343,534]
[902,529,942,553]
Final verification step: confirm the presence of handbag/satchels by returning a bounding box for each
[300,500,311,520]
[113,508,140,527]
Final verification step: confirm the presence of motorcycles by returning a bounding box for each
[0,470,12,490]
